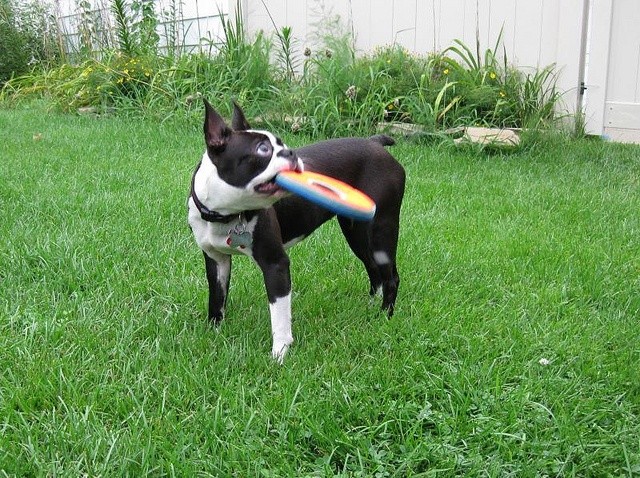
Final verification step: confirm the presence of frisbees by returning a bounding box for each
[276,170,376,222]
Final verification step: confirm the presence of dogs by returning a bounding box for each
[186,97,406,366]
